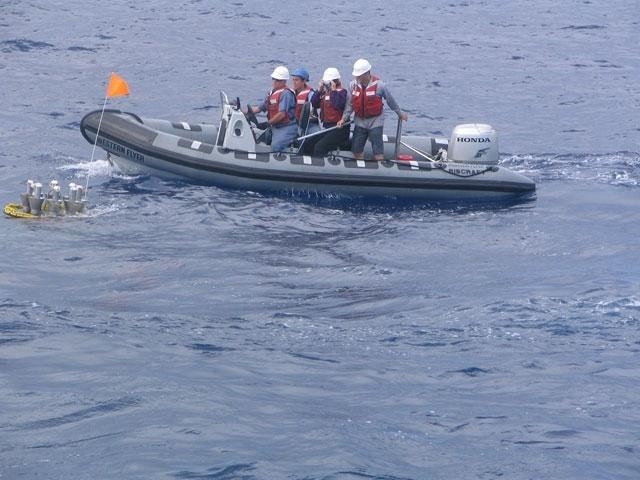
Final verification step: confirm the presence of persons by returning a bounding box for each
[291,69,321,134]
[299,68,352,158]
[338,58,409,160]
[234,65,299,152]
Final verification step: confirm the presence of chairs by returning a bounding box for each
[286,101,313,153]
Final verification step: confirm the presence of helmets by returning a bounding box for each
[290,66,311,82]
[352,58,372,78]
[323,67,340,81]
[270,65,291,81]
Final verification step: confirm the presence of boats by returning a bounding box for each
[80,90,536,201]
